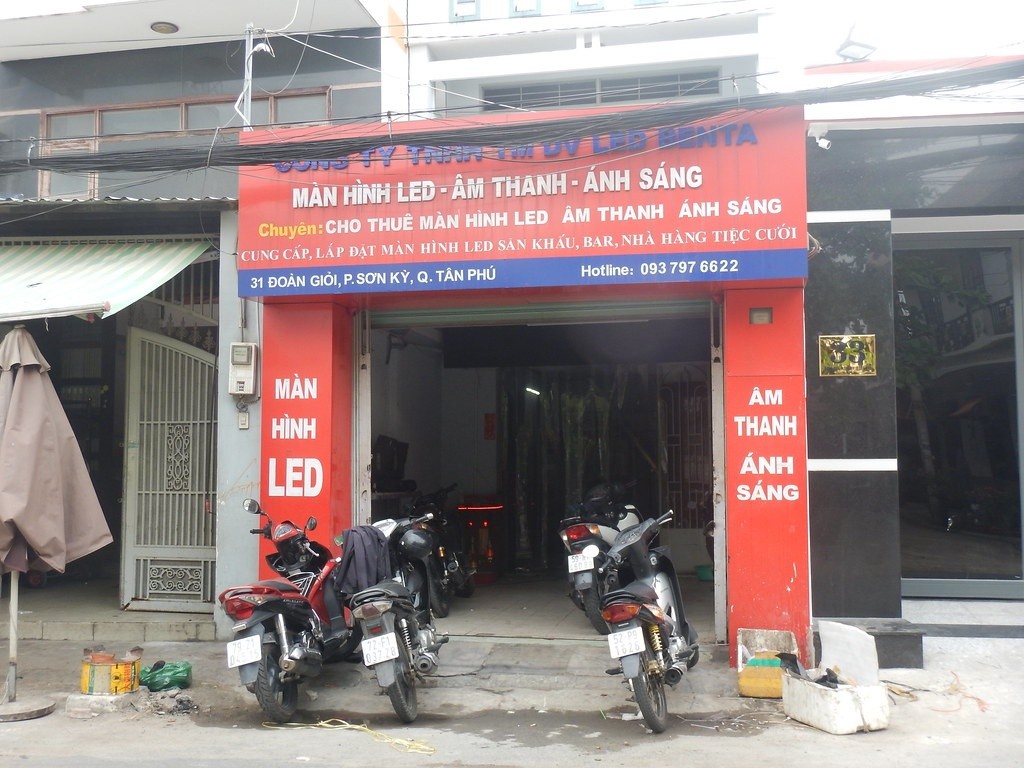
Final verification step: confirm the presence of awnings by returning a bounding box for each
[0,241,213,319]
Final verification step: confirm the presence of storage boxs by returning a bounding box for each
[782,669,890,735]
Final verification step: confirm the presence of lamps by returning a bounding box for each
[151,22,178,34]
[837,40,875,61]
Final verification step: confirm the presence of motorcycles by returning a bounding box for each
[218,483,476,724]
[558,481,699,734]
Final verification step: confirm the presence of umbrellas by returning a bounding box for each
[0,320,114,704]
